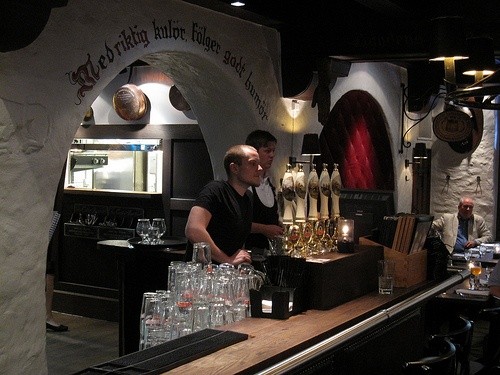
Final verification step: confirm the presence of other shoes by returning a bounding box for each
[46,322,68,332]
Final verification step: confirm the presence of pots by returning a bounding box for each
[169,84,191,113]
[112,65,148,121]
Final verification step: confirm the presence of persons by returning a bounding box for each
[47,260,68,332]
[185,144,263,269]
[245,130,284,250]
[431,196,493,254]
[474,261,500,375]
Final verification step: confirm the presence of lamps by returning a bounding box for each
[427,14,496,78]
[301,133,322,174]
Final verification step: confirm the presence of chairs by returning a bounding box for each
[383,294,500,375]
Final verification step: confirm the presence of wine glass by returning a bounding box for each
[471,261,482,283]
[136,217,167,244]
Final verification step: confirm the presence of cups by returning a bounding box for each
[377,259,396,294]
[139,241,265,350]
